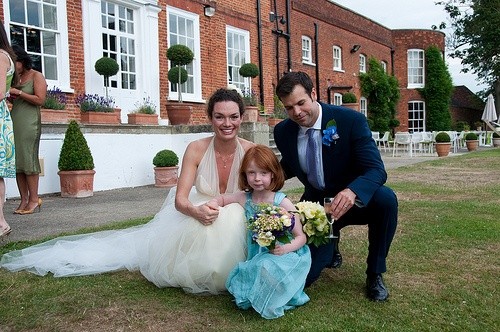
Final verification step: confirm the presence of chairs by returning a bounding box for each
[372,131,464,158]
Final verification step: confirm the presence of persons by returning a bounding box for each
[0,20,15,237]
[204,143,313,319]
[8,45,49,215]
[140,87,256,293]
[271,72,400,303]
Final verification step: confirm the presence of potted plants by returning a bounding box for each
[268,95,289,127]
[239,63,259,122]
[341,92,359,111]
[492,131,500,147]
[127,96,159,125]
[464,126,470,132]
[164,45,194,126]
[464,133,479,152]
[153,149,179,187]
[57,120,96,198]
[95,57,121,124]
[474,122,483,130]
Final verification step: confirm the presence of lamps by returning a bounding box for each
[269,12,286,25]
[353,44,360,52]
[204,5,215,17]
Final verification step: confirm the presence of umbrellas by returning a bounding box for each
[481,94,497,147]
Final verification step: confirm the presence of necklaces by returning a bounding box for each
[17,71,25,85]
[215,146,238,168]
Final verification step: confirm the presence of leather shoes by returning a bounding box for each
[332,230,342,269]
[365,272,390,302]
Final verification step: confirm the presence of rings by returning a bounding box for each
[343,205,348,208]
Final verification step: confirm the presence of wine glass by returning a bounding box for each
[324,198,338,238]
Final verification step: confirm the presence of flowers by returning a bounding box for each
[42,85,68,110]
[293,200,330,248]
[246,206,295,250]
[241,87,258,106]
[322,119,339,146]
[74,93,116,113]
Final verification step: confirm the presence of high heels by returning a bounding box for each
[0,224,12,239]
[15,197,42,214]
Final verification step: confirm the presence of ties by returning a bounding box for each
[306,129,320,192]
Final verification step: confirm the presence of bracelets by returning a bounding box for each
[18,90,22,97]
